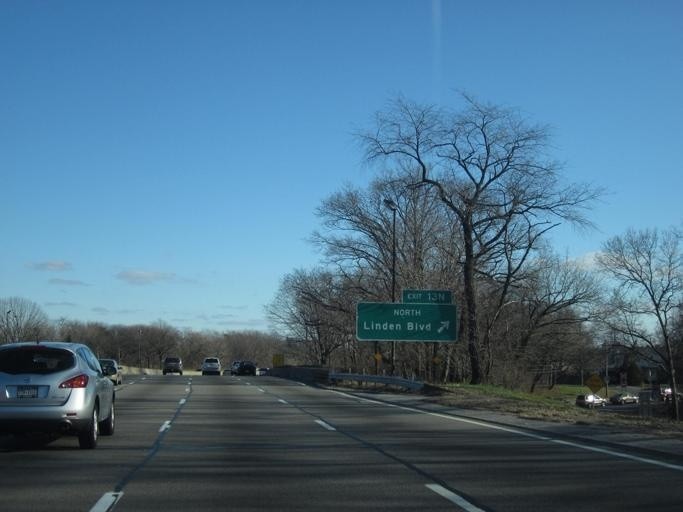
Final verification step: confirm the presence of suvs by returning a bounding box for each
[230,360,256,376]
[576,393,607,410]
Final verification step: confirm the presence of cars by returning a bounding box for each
[201,357,220,375]
[610,391,640,404]
[161,356,183,376]
[0,341,117,449]
[96,358,123,387]
[195,367,201,371]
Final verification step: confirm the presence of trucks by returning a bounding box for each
[222,370,231,376]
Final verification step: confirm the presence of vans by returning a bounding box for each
[649,387,682,401]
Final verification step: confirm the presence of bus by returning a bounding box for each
[255,368,268,376]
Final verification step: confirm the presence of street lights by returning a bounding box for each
[5,309,10,345]
[379,198,398,377]
[601,339,610,396]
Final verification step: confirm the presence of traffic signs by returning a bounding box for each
[401,289,451,305]
[357,301,456,344]
